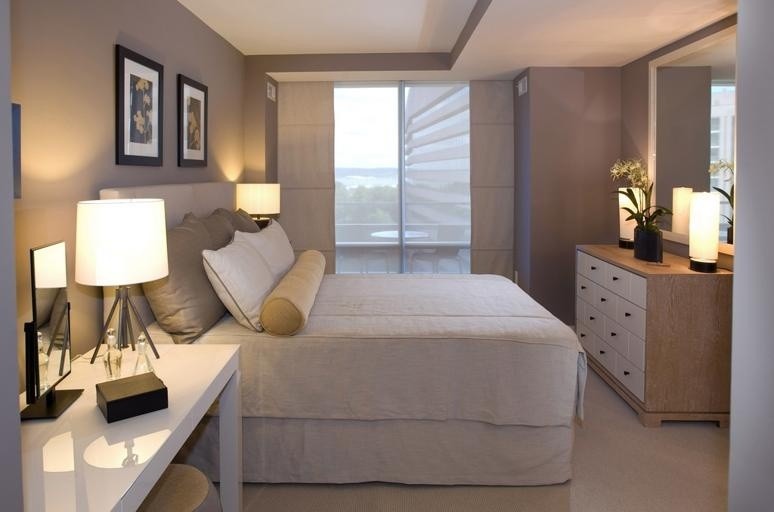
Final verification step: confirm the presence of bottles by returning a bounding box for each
[102,328,123,378]
[36,331,50,395]
[133,334,153,376]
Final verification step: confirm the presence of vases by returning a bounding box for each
[632,226,664,263]
[725,226,733,244]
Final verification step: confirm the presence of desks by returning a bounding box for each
[17,342,246,512]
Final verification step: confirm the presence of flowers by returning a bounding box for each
[606,155,674,232]
[707,159,733,226]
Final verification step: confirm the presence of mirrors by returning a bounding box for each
[644,24,739,256]
[18,239,88,421]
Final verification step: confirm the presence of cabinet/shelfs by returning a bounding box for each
[574,245,732,429]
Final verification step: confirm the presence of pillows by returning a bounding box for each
[234,217,296,284]
[141,209,228,344]
[185,208,237,250]
[258,249,326,335]
[200,229,278,333]
[218,206,261,232]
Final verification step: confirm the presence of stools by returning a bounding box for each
[136,462,225,511]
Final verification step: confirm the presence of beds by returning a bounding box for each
[98,180,586,484]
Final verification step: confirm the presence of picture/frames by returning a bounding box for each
[176,74,209,167]
[113,42,164,166]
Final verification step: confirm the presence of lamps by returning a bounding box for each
[615,187,643,250]
[687,191,720,272]
[671,187,693,234]
[71,199,170,365]
[234,181,280,221]
[34,241,70,376]
